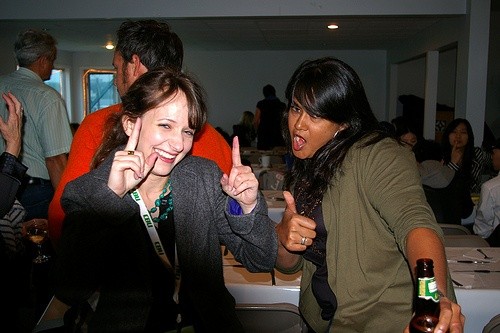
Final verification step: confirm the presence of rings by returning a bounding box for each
[127,149,134,154]
[301,236,307,245]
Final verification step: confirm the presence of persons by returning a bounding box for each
[397,116,499,242]
[276,57,465,333]
[66,118,85,138]
[252,85,286,146]
[48,17,233,235]
[232,110,255,147]
[0,27,73,290]
[48,66,275,332]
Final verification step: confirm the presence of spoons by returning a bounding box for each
[477,248,493,260]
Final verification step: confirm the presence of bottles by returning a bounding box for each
[409,258,450,333]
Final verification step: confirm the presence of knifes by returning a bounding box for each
[453,270,490,273]
[446,260,473,264]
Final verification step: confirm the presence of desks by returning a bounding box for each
[224,147,500,333]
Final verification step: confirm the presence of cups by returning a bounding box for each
[258,156,270,167]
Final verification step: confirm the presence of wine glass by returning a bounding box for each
[22,219,49,264]
[472,194,481,209]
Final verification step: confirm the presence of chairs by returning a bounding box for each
[236,303,300,333]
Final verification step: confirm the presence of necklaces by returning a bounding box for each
[147,176,174,223]
